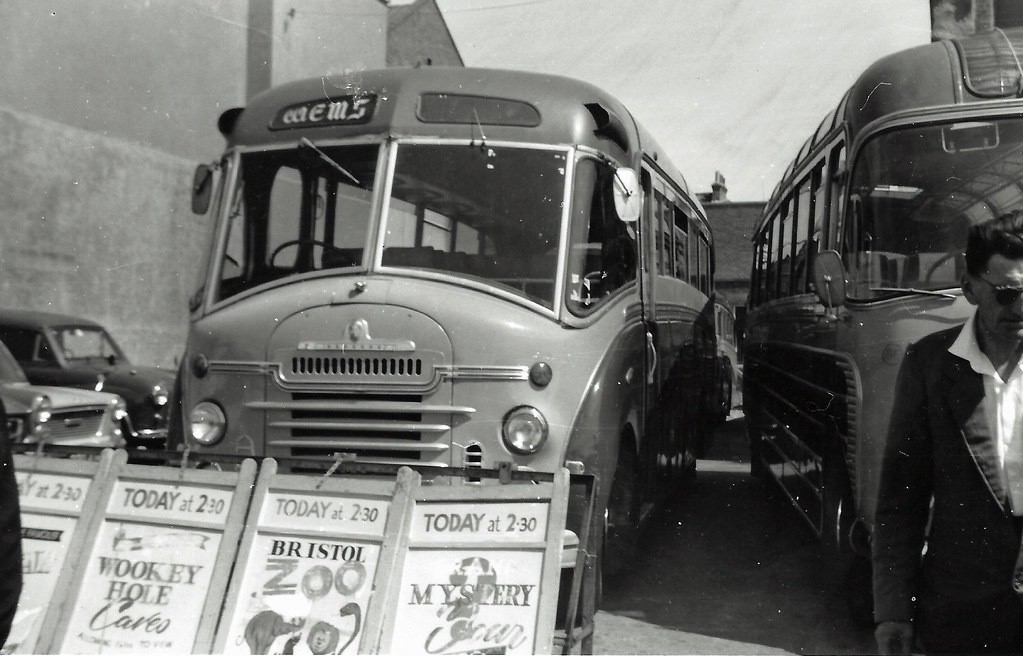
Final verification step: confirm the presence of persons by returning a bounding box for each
[872,211,1022,656]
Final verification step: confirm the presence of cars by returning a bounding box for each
[0,341,127,461]
[0,306,179,465]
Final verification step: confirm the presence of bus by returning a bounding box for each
[161,58,721,629]
[714,291,744,422]
[729,23,1023,624]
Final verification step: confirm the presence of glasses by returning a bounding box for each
[979,275,1023,305]
